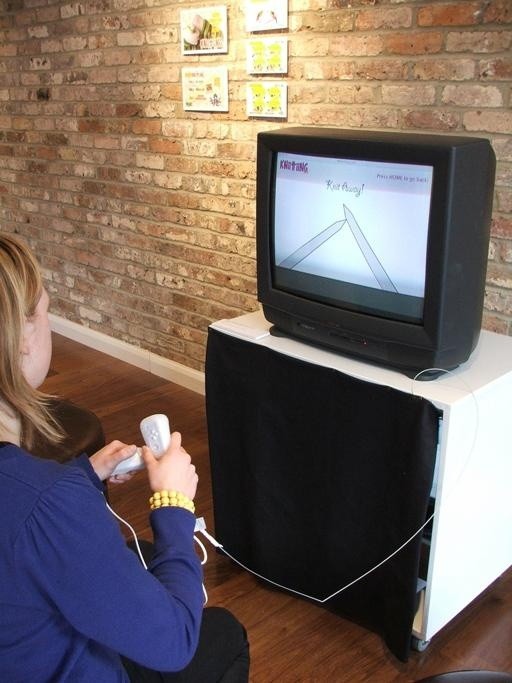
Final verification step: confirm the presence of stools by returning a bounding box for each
[37,400,110,505]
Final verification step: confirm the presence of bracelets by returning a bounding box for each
[148,490,196,514]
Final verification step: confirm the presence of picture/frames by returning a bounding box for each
[181,0,288,121]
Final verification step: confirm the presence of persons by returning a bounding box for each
[0,228,250,683]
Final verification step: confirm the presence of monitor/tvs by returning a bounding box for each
[257,127,496,374]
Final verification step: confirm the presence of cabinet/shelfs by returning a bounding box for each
[203,308,510,646]
